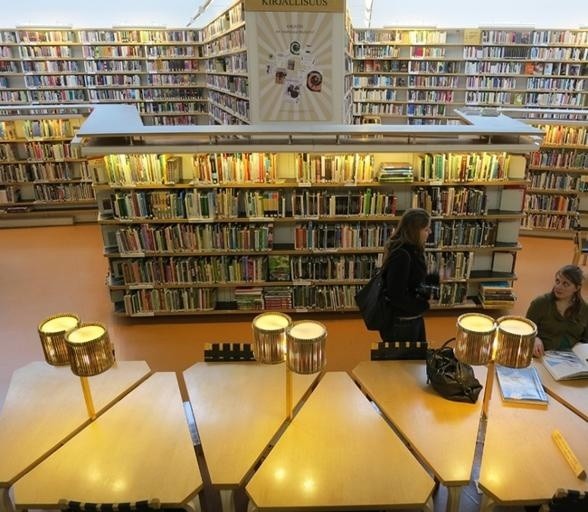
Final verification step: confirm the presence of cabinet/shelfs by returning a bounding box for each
[71,104,546,319]
[345,27,587,254]
[1,0,246,212]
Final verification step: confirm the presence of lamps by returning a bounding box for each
[36,310,116,421]
[251,311,329,422]
[452,313,539,418]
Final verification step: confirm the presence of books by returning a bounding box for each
[542,342,588,382]
[496,363,549,405]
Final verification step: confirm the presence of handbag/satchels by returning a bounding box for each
[353,268,392,331]
[426,337,483,404]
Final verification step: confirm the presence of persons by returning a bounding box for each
[378,208,446,342]
[526,264,588,358]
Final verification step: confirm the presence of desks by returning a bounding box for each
[183,361,436,512]
[351,361,588,512]
[1,359,202,511]
[532,357,587,420]
